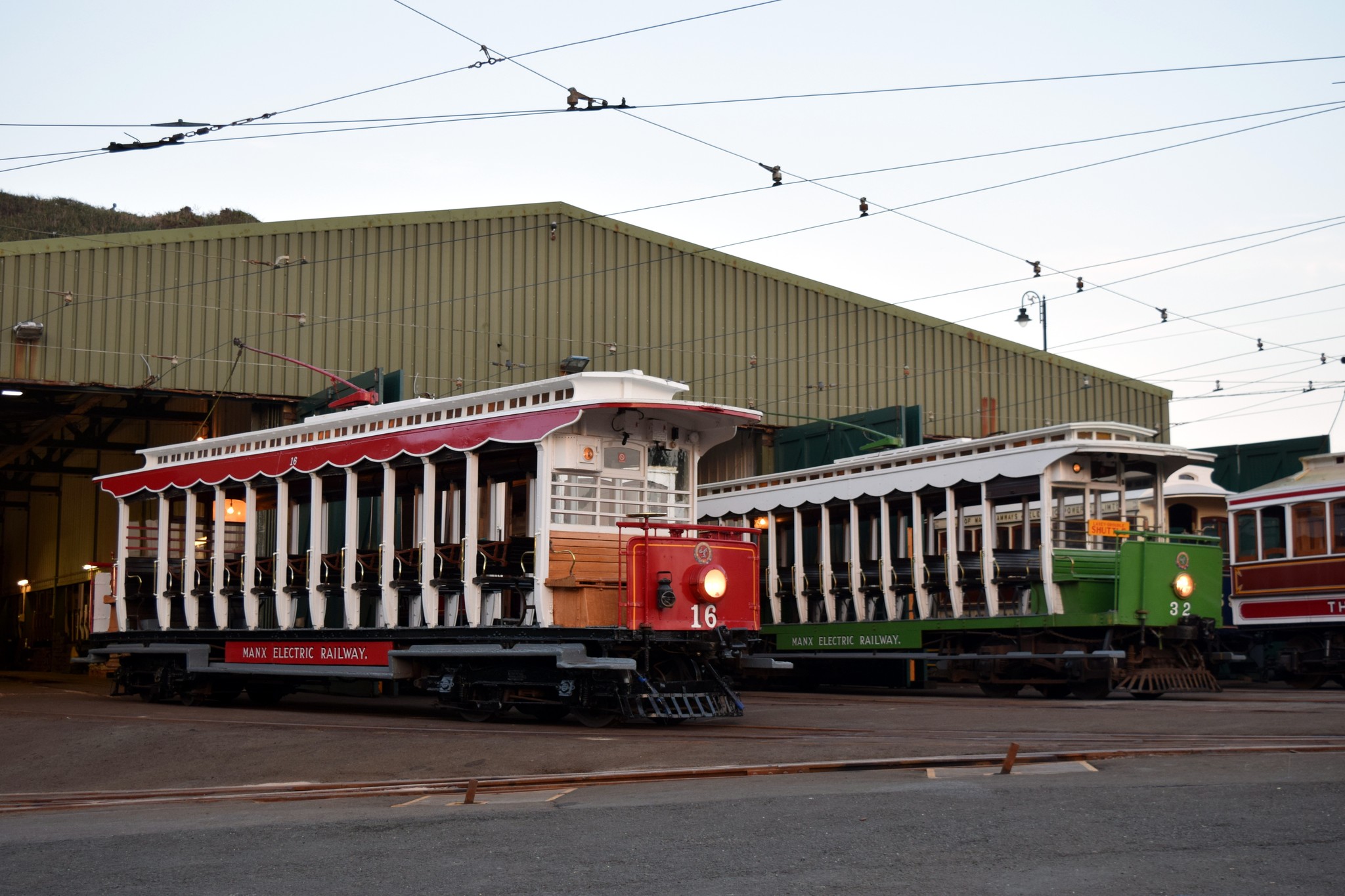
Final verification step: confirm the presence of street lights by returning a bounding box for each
[1014,291,1048,355]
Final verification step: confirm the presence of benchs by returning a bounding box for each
[760,548,1120,618]
[123,536,535,625]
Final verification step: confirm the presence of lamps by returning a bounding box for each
[13,321,43,343]
[560,355,590,373]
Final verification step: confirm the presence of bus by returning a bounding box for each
[686,421,1232,703]
[71,336,770,719]
[1228,452,1345,689]
